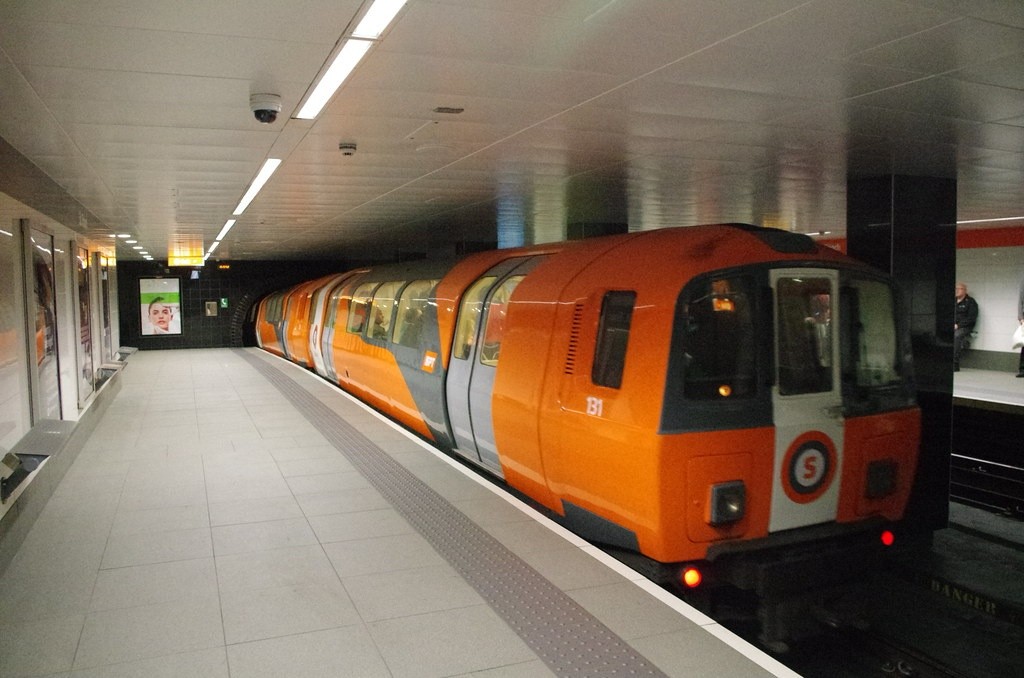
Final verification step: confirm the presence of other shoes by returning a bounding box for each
[953,363,959,372]
[1016,374,1024,378]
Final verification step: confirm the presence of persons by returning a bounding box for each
[482,288,505,359]
[955,282,978,371]
[803,293,830,369]
[1014,277,1024,377]
[353,291,430,347]
[148,297,173,332]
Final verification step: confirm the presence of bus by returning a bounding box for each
[254,222,925,593]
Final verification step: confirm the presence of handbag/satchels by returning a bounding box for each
[1012,323,1024,349]
